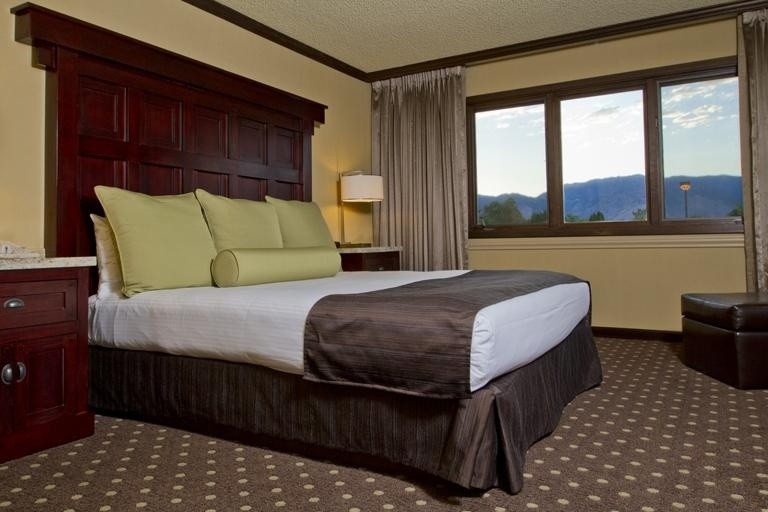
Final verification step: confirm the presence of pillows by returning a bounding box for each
[87,184,342,301]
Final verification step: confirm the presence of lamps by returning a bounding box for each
[334,169,384,248]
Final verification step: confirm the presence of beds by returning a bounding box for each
[83,271,594,494]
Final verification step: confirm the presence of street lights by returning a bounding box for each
[680,182,691,217]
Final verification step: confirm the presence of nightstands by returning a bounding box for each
[1,255,96,464]
[340,246,404,271]
